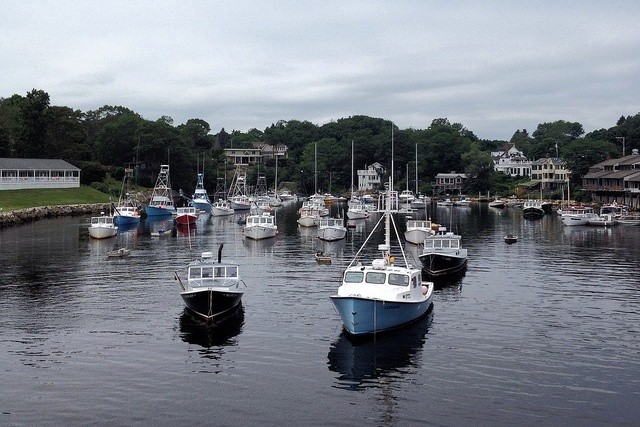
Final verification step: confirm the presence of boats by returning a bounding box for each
[315,250,331,262]
[330,177,434,335]
[250,199,272,215]
[297,207,323,226]
[487,198,504,208]
[399,164,415,201]
[347,199,371,220]
[88,216,118,239]
[299,200,329,216]
[586,213,618,228]
[175,244,247,321]
[319,218,347,240]
[146,164,175,215]
[324,193,336,200]
[600,201,624,223]
[418,227,468,277]
[270,193,281,206]
[280,190,294,200]
[436,194,453,206]
[231,195,251,209]
[404,220,435,244]
[309,143,326,200]
[176,207,197,224]
[561,209,589,225]
[557,179,594,215]
[348,140,365,205]
[246,213,277,239]
[504,234,517,242]
[411,199,426,208]
[192,174,211,211]
[211,199,234,216]
[339,196,346,200]
[108,247,130,257]
[113,169,141,224]
[621,214,640,228]
[542,201,552,210]
[523,200,545,219]
[454,195,471,205]
